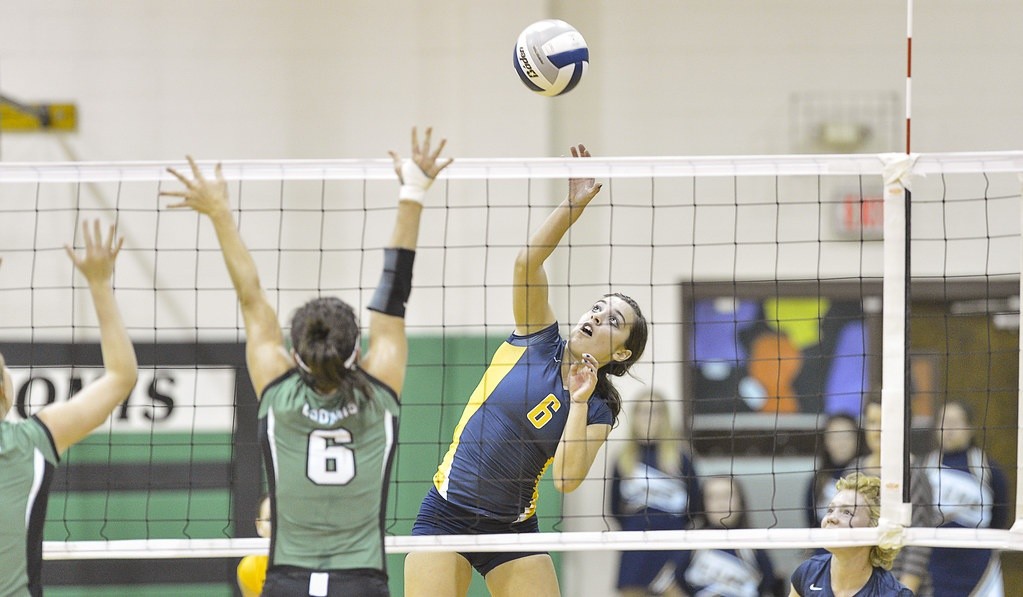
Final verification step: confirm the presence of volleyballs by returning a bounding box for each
[512,17,591,98]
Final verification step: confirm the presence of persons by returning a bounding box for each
[0,219,138,597]
[789,473,914,597]
[923,402,1008,597]
[159,126,453,597]
[237,493,272,597]
[404,145,647,597]
[805,393,934,597]
[609,392,775,597]
